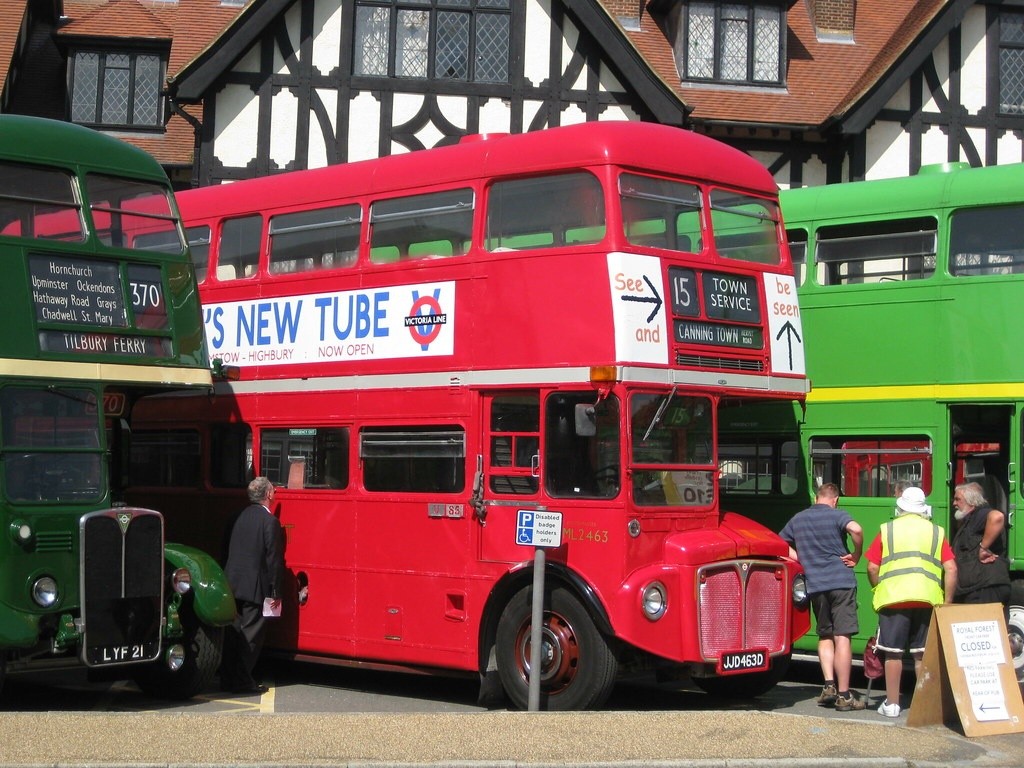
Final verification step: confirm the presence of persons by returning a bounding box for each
[222,476,286,694]
[952,481,1013,604]
[777,483,866,712]
[862,479,960,718]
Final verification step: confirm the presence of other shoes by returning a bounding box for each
[231,681,268,692]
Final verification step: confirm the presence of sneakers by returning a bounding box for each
[836,694,865,710]
[813,685,838,706]
[877,699,901,716]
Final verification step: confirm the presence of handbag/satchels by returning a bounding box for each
[244,568,271,653]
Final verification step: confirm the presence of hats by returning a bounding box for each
[895,487,929,515]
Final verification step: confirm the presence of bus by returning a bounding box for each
[0,122,822,716]
[355,156,1024,684]
[1,112,275,702]
